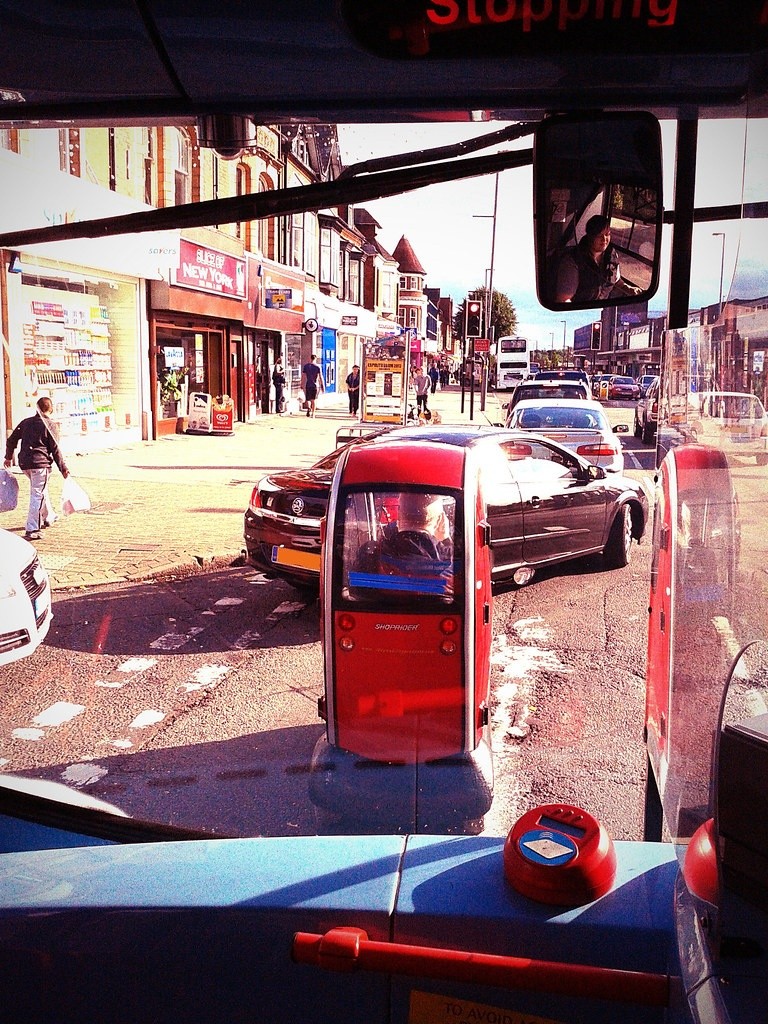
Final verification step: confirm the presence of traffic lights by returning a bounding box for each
[465,299,482,338]
[591,322,602,350]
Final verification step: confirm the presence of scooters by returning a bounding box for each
[317,440,493,764]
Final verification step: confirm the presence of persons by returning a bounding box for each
[681,500,718,586]
[345,365,360,415]
[411,366,451,417]
[274,358,284,413]
[3,397,71,539]
[354,494,456,602]
[300,354,324,418]
[562,214,642,303]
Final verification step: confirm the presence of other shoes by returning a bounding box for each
[349,411,356,416]
[306,407,311,417]
[25,530,44,540]
[43,515,58,527]
[311,414,316,418]
[276,410,284,413]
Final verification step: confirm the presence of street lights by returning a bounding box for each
[712,231,725,316]
[485,267,495,340]
[550,332,555,365]
[561,321,569,367]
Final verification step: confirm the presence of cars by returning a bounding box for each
[491,398,629,476]
[0,526,50,665]
[244,426,649,591]
[502,362,768,467]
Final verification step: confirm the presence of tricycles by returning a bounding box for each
[643,443,741,837]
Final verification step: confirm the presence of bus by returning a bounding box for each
[489,335,530,392]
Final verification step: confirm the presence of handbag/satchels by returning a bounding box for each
[61,474,90,516]
[0,467,19,513]
